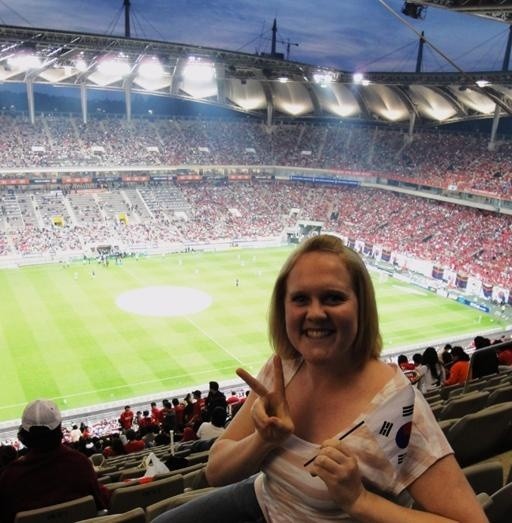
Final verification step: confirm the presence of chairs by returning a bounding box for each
[0,186,192,230]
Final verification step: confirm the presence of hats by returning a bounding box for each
[21,398,63,433]
[192,390,202,395]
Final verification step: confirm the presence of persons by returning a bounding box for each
[396,332,512,393]
[148,232,493,523]
[1,105,512,319]
[1,380,252,522]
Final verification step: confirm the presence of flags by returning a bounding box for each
[362,383,416,491]
